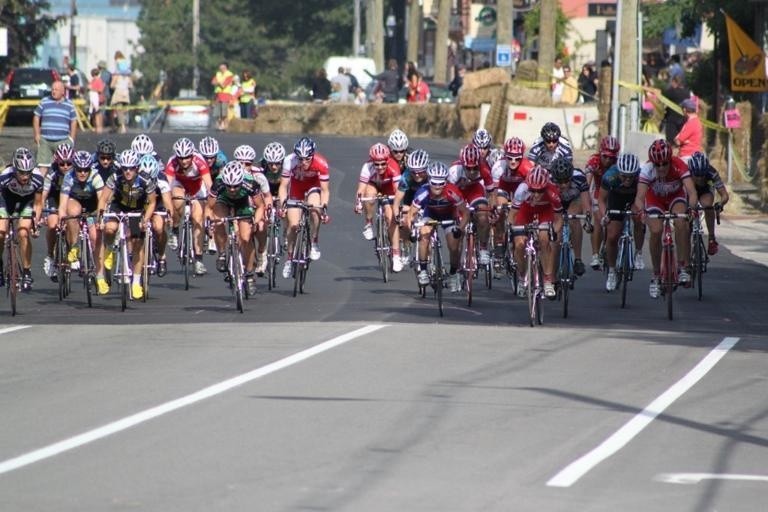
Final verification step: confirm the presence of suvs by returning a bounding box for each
[60,67,91,119]
[2,67,68,127]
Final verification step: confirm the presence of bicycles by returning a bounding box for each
[281,199,329,299]
[601,203,643,308]
[558,209,592,319]
[61,207,101,309]
[204,207,258,314]
[512,214,558,328]
[172,194,208,291]
[687,203,722,300]
[642,208,693,321]
[358,191,400,283]
[98,210,149,313]
[408,198,512,316]
[41,208,73,301]
[0,211,42,317]
[266,196,284,293]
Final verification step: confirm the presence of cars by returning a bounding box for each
[398,83,458,103]
[160,89,216,132]
[115,92,163,130]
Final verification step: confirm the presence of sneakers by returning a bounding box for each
[606,273,617,291]
[21,246,167,300]
[476,250,491,265]
[449,275,458,293]
[573,260,585,276]
[493,258,504,280]
[707,240,718,254]
[517,281,527,298]
[392,255,403,271]
[362,223,374,240]
[167,235,321,295]
[677,268,690,284]
[589,256,600,268]
[543,282,556,297]
[634,253,644,270]
[417,270,430,285]
[649,278,661,299]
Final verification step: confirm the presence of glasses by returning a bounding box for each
[654,161,668,167]
[529,187,545,195]
[430,180,446,187]
[603,154,617,160]
[545,139,558,143]
[466,166,479,171]
[373,162,388,167]
[620,172,638,178]
[392,149,406,154]
[506,155,524,162]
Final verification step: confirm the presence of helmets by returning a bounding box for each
[649,139,673,162]
[600,135,620,155]
[369,129,449,189]
[552,156,574,181]
[542,122,561,140]
[504,136,524,155]
[473,128,492,149]
[53,134,316,186]
[688,152,710,173]
[462,143,480,167]
[13,147,36,172]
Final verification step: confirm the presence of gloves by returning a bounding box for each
[452,227,462,239]
[30,227,40,238]
[354,203,363,214]
[320,214,329,224]
[685,208,698,223]
[277,208,288,218]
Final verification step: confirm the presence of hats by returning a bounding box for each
[679,99,696,108]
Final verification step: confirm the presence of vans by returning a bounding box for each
[323,54,378,91]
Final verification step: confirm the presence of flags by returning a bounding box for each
[724,14,768,93]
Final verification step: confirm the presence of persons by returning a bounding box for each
[355,124,729,299]
[548,48,703,122]
[208,62,270,131]
[0,134,331,300]
[33,80,76,179]
[0,50,168,124]
[305,48,490,105]
